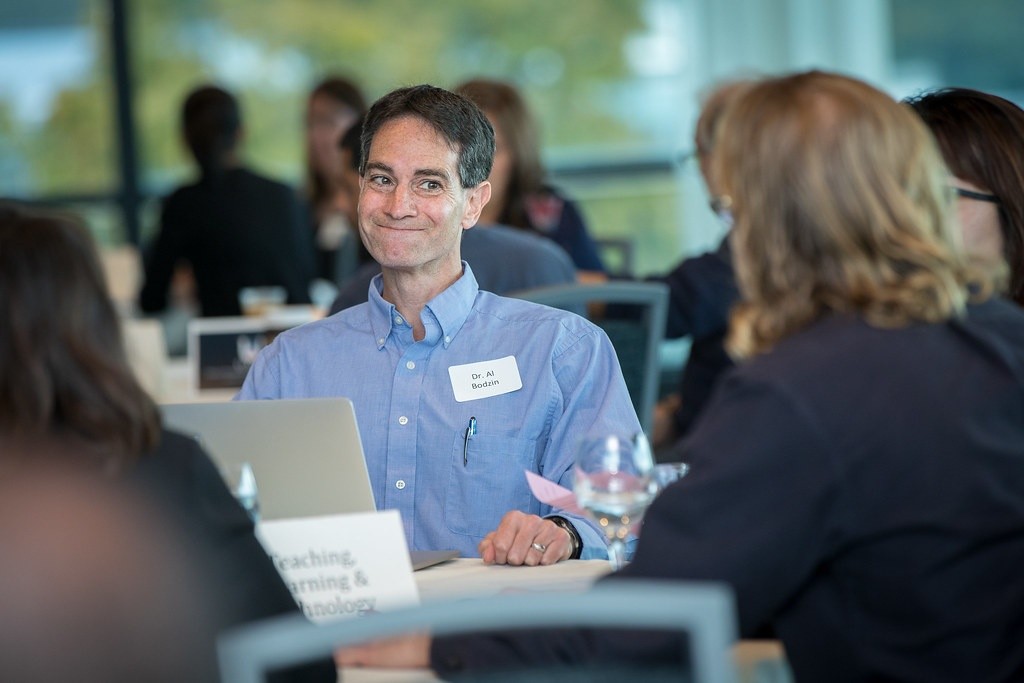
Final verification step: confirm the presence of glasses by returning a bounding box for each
[710,199,733,223]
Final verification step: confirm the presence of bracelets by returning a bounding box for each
[543,515,583,560]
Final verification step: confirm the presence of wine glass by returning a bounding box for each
[572,432,657,573]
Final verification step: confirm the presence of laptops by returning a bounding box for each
[155,398,460,576]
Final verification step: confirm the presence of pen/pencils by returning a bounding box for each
[462,416,479,467]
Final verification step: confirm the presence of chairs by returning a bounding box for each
[218,577,742,683]
[506,283,670,449]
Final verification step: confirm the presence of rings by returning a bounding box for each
[531,542,548,554]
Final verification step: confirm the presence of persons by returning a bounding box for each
[132,78,605,318]
[0,199,338,683]
[233,85,653,567]
[586,71,1024,683]
